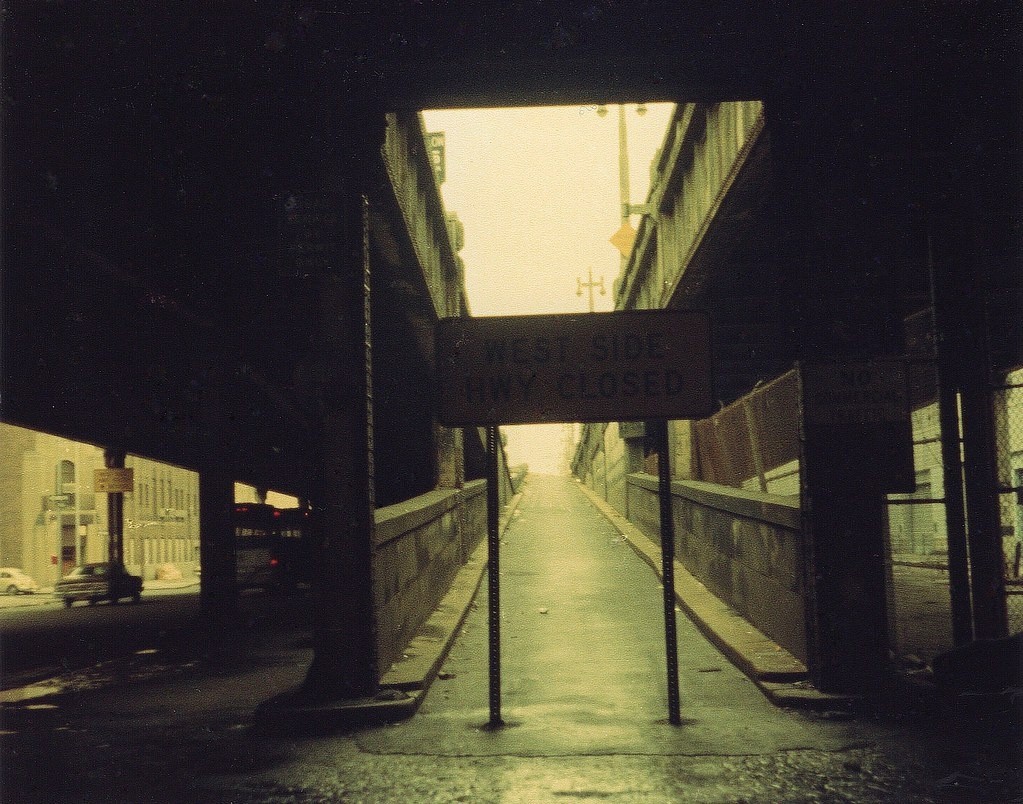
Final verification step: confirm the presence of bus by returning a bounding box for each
[232,501,310,594]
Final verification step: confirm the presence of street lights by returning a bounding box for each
[596,102,646,271]
[576,279,607,313]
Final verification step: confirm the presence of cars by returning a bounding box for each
[54,564,146,607]
[1,567,41,596]
